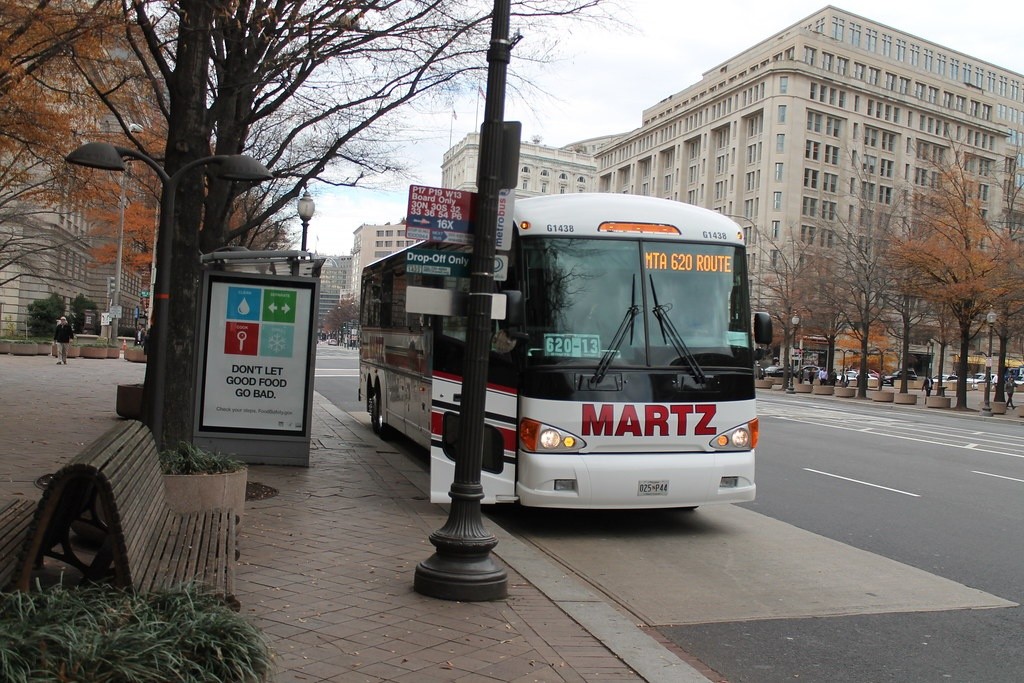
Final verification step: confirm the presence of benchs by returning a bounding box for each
[0,419,241,618]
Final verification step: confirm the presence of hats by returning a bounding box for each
[61,317,66,319]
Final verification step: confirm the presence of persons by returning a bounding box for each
[134,325,145,347]
[54,317,75,365]
[993,373,1018,410]
[346,333,359,351]
[756,366,885,388]
[922,373,934,404]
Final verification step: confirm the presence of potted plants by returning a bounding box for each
[158,440,248,518]
[0,315,147,363]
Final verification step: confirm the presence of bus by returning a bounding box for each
[359,194,774,512]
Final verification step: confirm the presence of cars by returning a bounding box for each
[761,365,1024,387]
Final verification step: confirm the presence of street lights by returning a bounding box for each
[297,186,315,263]
[870,347,894,392]
[65,141,274,463]
[979,304,997,416]
[786,310,799,393]
[834,348,858,387]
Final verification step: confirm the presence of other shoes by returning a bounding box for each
[1011,405,1016,410]
[56,362,68,365]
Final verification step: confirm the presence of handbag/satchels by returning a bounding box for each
[992,385,994,389]
